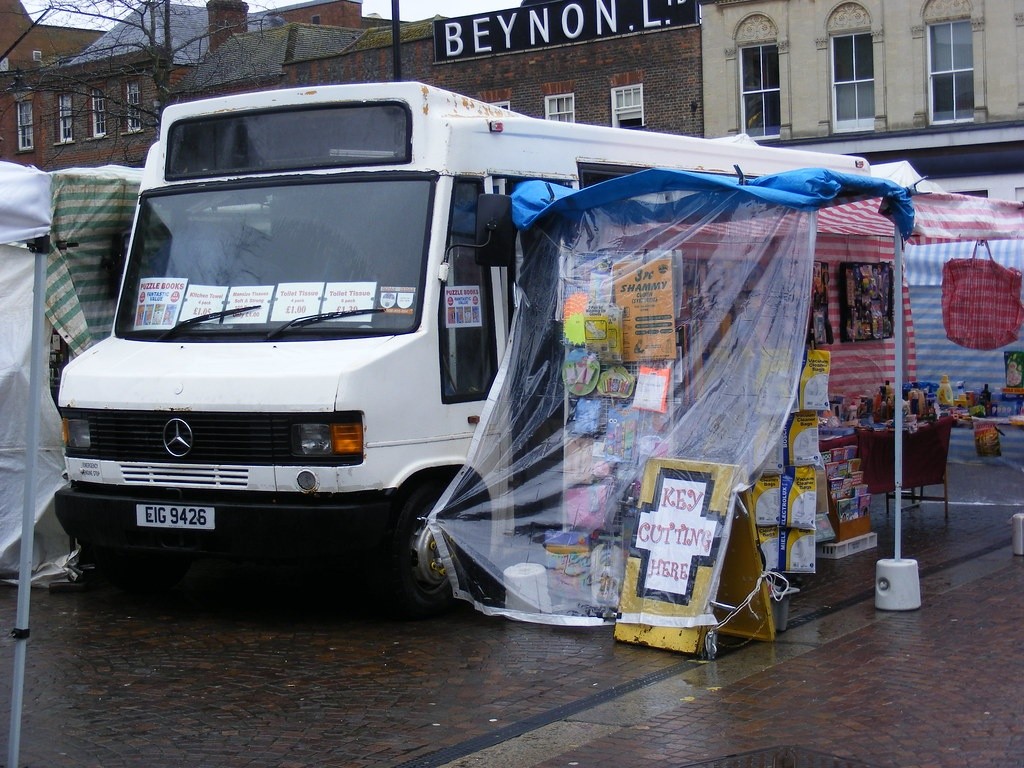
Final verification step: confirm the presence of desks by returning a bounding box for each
[819,415,956,521]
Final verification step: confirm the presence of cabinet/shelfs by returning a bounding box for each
[825,483,871,542]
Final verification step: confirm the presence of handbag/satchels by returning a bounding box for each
[941,239,1024,351]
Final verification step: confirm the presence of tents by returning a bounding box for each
[0,162,51,767]
[510,169,1024,611]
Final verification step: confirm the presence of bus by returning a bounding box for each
[47,79,873,614]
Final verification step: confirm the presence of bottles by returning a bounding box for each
[817,375,1024,437]
